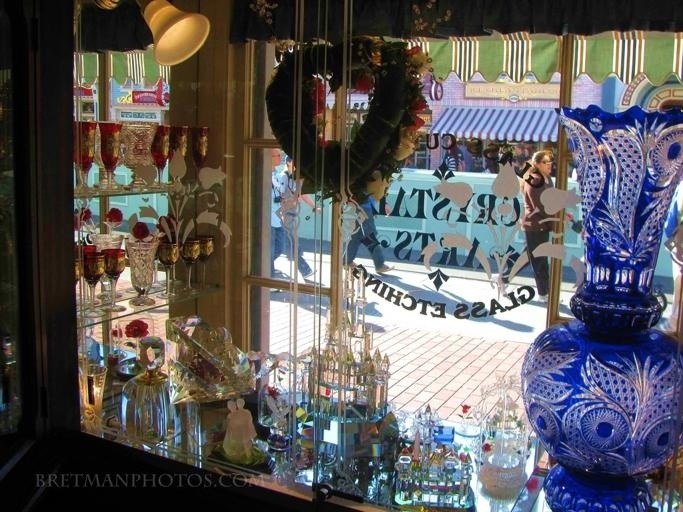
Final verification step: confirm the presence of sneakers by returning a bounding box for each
[538,294,566,304]
[302,269,318,280]
[493,276,508,297]
[343,261,360,270]
[376,265,396,273]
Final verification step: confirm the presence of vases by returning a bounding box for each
[521,104,682,512]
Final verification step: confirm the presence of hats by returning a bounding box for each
[285,155,294,164]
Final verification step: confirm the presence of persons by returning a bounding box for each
[270,139,550,303]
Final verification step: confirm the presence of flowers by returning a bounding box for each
[264,36,433,204]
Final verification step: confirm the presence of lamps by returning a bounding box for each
[89,0,211,66]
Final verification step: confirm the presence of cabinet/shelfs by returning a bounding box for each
[74,178,223,410]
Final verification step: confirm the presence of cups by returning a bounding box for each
[81,365,107,418]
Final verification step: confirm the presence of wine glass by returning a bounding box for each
[74,117,209,198]
[76,230,215,316]
